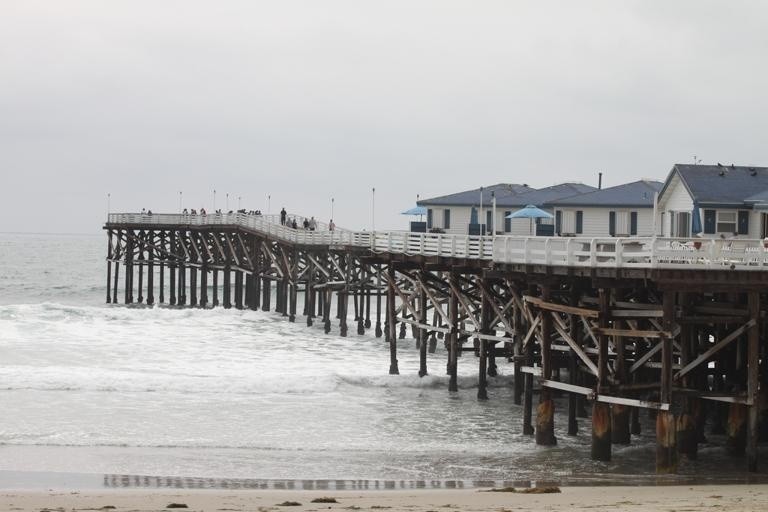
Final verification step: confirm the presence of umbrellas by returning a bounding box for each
[506,203,554,237]
[400,205,430,222]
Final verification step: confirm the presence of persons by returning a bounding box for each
[139,206,336,237]
[718,231,739,247]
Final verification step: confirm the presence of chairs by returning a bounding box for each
[669,240,768,266]
